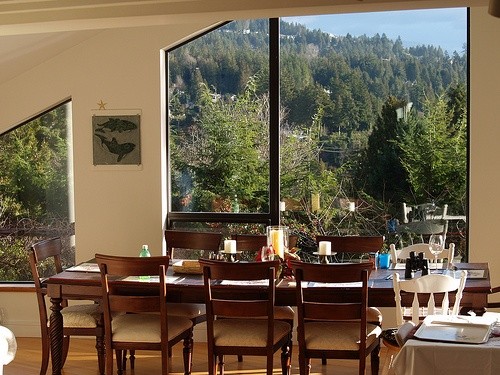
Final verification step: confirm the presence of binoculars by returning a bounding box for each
[405,251,429,279]
[407,206,426,222]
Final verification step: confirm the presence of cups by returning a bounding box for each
[267,225,289,261]
[261,246,274,262]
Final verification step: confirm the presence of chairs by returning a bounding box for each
[28,230,500,375]
[403,203,448,248]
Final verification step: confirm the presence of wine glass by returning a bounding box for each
[429,235,444,273]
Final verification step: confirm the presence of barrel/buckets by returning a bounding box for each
[379,253,391,269]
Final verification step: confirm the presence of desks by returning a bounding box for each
[41,257,492,375]
[388,315,500,375]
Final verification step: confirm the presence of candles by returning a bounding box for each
[279,201,286,211]
[270,230,285,261]
[349,202,355,213]
[224,240,236,253]
[319,241,331,255]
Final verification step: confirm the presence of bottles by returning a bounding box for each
[138,244,151,279]
[405,251,430,280]
